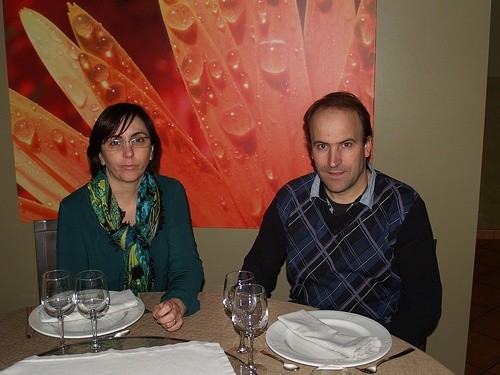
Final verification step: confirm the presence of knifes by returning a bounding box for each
[26,306,35,339]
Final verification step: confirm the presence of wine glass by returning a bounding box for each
[76,270,111,353]
[40,269,76,355]
[222,271,260,363]
[231,283,269,375]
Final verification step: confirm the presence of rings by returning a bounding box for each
[174,319,177,324]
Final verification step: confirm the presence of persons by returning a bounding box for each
[56,102,204,332]
[238,91,443,347]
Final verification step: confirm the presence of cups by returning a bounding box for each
[309,364,352,375]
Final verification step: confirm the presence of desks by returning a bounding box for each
[0,292,455,375]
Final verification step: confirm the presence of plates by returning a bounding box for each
[265,310,393,368]
[28,290,145,339]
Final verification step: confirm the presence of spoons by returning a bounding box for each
[80,329,131,344]
[260,350,300,371]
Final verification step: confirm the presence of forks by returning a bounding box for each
[356,347,415,374]
[136,292,153,316]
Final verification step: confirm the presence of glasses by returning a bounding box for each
[102,137,152,151]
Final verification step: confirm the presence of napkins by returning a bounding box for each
[277,309,381,359]
[40,289,138,322]
